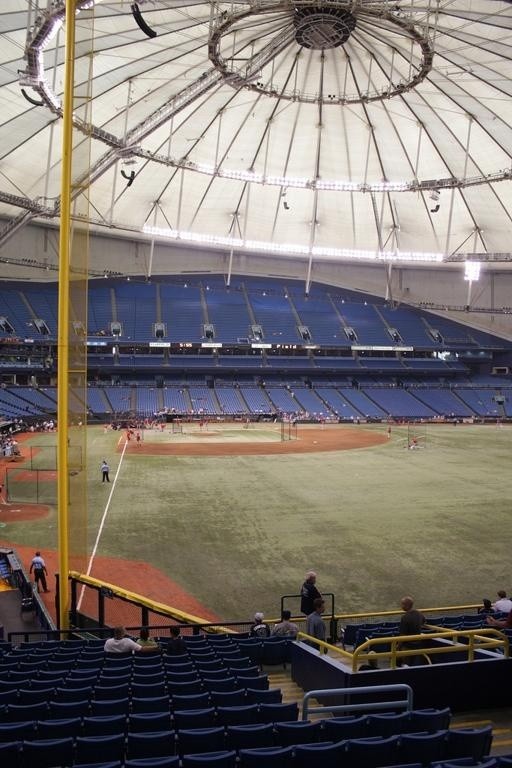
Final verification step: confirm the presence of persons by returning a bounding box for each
[359,590,512,671]
[162,406,204,414]
[0,484,4,493]
[104,624,160,653]
[167,626,187,655]
[273,610,300,637]
[102,410,165,446]
[101,461,110,481]
[411,438,417,445]
[434,412,457,426]
[300,572,328,654]
[273,409,360,427]
[199,420,209,432]
[29,551,51,592]
[136,627,157,647]
[251,612,271,638]
[387,426,391,438]
[0,418,54,463]
